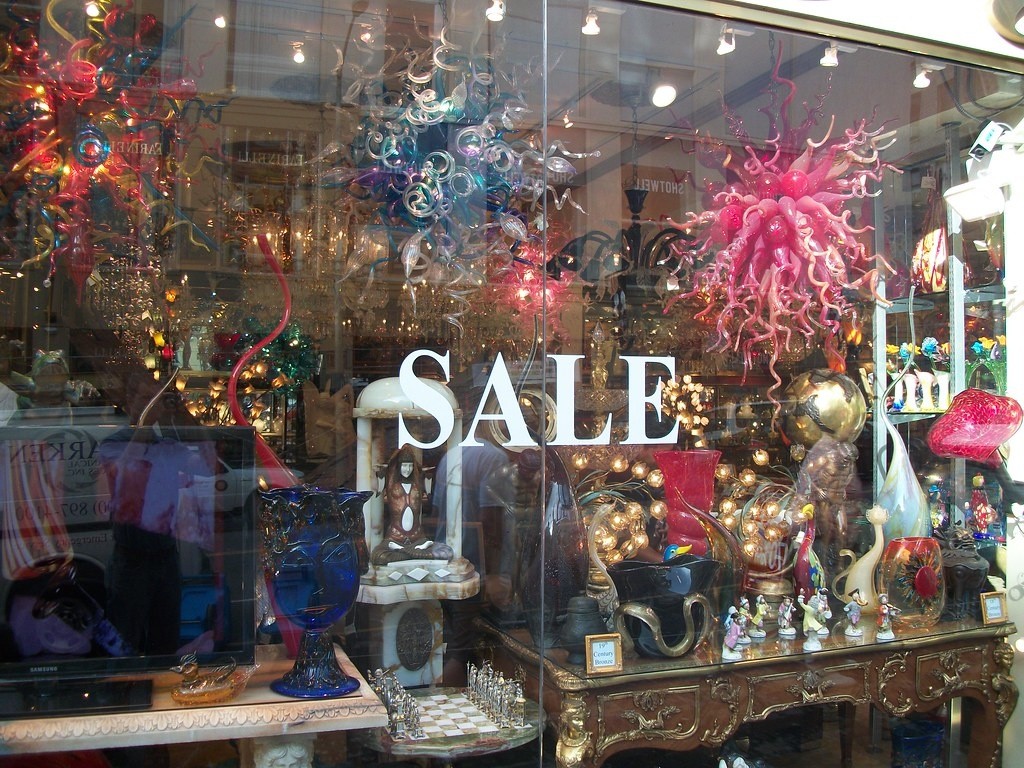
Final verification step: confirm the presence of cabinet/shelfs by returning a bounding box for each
[872,121,1024,533]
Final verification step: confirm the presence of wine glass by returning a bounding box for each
[256,487,372,701]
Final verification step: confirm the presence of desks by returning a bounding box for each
[0,640,389,768]
[474,593,1022,768]
[356,686,547,768]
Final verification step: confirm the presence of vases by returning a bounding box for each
[888,360,1007,410]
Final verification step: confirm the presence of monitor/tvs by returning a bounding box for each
[0,424,256,719]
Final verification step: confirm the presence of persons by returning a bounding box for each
[371,446,453,563]
[721,588,897,660]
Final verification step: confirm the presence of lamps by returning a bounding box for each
[535,80,716,305]
[292,0,947,109]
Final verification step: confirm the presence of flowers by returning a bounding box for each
[886,335,1007,370]
